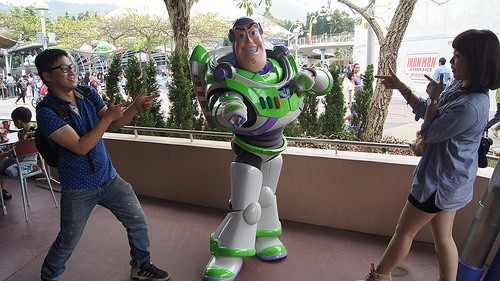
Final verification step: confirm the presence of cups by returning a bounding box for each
[0,129,7,140]
[1,120,11,131]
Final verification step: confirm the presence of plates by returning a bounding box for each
[0,138,9,143]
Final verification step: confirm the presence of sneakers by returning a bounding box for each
[130,260,170,281]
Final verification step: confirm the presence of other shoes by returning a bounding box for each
[2,189,11,200]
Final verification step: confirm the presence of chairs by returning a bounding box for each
[0,135,58,222]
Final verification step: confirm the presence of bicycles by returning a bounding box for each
[30,84,44,109]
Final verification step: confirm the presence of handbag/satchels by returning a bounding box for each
[478,136,493,168]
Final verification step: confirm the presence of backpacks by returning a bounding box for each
[34,85,101,173]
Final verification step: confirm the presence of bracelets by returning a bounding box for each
[399,86,412,105]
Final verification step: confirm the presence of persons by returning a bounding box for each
[337,61,364,132]
[0,72,43,105]
[79,71,132,100]
[431,56,452,90]
[482,88,500,138]
[34,48,170,281]
[189,17,333,281]
[0,107,38,202]
[364,29,500,281]
[160,67,174,85]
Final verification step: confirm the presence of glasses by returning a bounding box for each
[47,64,76,73]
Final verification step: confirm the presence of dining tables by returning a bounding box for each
[0,132,28,146]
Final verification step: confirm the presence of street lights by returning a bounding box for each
[307,16,317,44]
[34,1,50,50]
[292,28,300,63]
